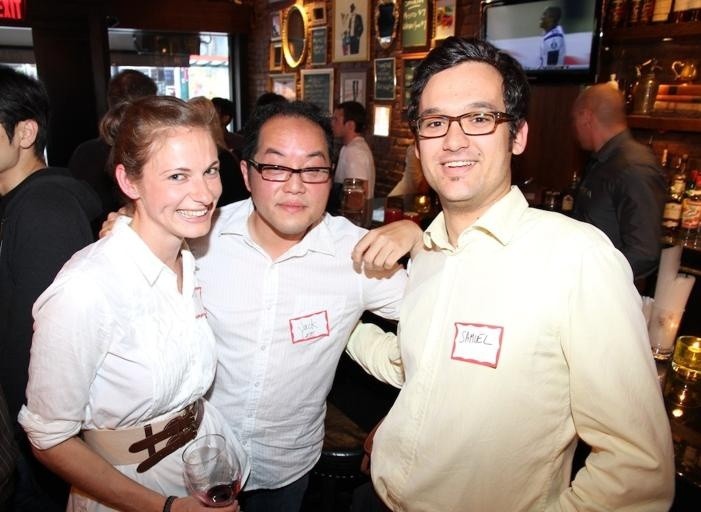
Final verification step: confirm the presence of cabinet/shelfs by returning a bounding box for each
[588,1,701,275]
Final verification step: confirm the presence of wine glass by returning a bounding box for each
[181,434,244,512]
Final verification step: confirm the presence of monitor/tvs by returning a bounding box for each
[479,0,602,87]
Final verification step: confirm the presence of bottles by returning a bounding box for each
[522,130,700,249]
[339,176,367,216]
[604,0,701,113]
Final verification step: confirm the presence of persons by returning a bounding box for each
[344,35,675,512]
[571,83,665,298]
[348,4,363,54]
[539,5,566,67]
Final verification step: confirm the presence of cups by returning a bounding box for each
[383,192,440,227]
[640,297,700,386]
[662,372,700,410]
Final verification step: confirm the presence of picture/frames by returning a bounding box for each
[373,57,396,101]
[336,69,370,111]
[399,1,432,52]
[268,43,284,73]
[300,68,335,116]
[269,12,282,41]
[431,0,457,40]
[372,105,392,138]
[309,26,329,66]
[268,72,297,103]
[331,1,370,64]
[309,2,328,25]
[400,54,427,113]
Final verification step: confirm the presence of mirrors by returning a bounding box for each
[282,4,307,68]
[373,1,399,50]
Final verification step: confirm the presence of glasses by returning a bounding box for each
[247,158,335,187]
[413,108,514,141]
